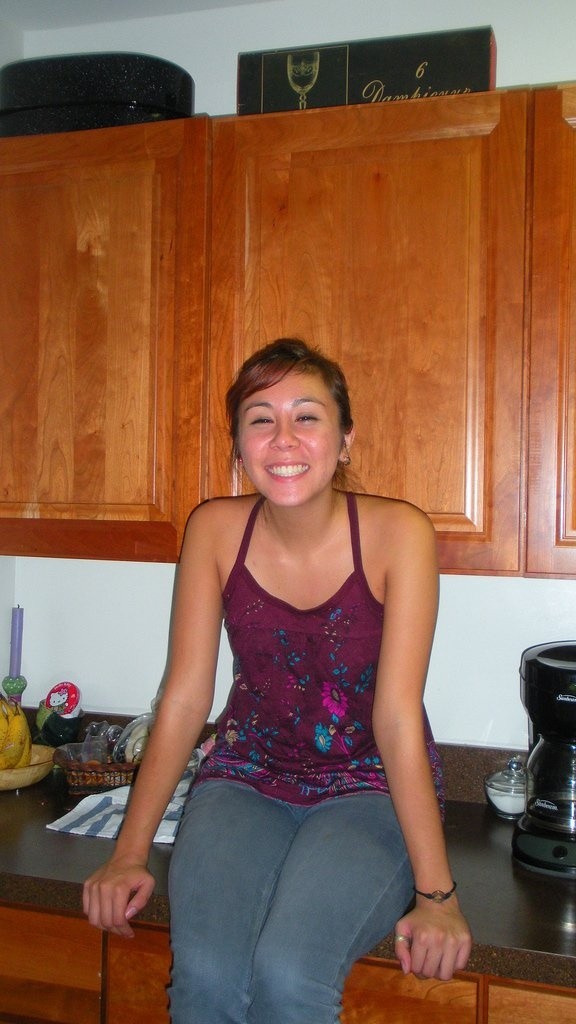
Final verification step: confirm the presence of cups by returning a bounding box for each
[36,700,53,730]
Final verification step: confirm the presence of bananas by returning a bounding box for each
[0,693,32,771]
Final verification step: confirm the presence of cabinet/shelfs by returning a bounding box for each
[0,903,576,1024]
[1,81,576,579]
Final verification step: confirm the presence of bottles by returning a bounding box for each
[485,757,526,820]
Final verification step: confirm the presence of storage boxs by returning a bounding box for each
[237,26,498,115]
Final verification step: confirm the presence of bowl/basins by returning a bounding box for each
[0,745,56,790]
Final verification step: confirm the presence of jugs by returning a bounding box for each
[523,731,576,836]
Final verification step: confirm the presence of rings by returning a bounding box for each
[392,934,411,945]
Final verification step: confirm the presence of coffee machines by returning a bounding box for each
[511,640,576,877]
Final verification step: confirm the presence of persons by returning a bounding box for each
[84,337,472,1024]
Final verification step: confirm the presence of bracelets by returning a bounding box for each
[411,880,458,903]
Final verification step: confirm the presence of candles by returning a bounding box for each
[10,604,24,678]
